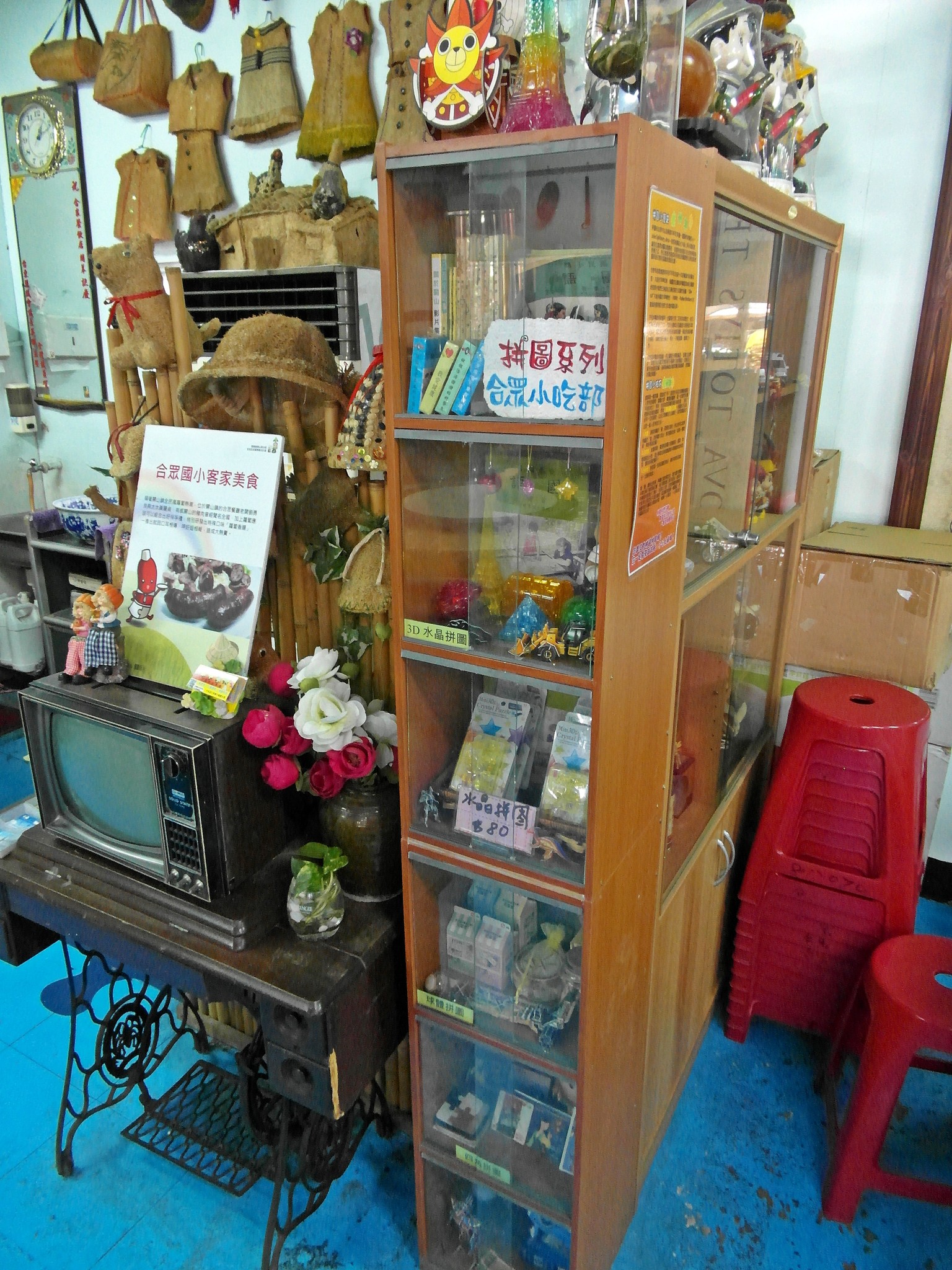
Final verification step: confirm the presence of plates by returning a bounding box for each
[403,484,558,526]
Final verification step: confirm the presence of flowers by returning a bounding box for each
[232,637,404,799]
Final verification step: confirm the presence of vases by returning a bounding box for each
[307,779,401,902]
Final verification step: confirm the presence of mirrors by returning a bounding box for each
[0,83,108,414]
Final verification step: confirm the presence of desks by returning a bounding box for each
[0,840,410,1270]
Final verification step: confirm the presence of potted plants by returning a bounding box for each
[275,841,354,950]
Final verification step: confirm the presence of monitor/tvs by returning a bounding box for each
[17,670,291,903]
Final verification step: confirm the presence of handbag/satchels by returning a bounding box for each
[92,1,170,117]
[31,0,103,81]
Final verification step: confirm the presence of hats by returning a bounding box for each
[177,312,351,439]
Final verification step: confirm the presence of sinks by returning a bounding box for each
[1,508,44,569]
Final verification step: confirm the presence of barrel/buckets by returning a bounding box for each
[0,592,45,673]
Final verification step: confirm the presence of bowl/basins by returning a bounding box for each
[52,492,119,545]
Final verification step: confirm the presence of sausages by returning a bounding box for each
[164,553,254,631]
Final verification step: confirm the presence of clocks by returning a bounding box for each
[13,89,66,180]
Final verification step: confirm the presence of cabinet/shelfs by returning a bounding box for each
[369,113,844,1270]
[32,534,112,674]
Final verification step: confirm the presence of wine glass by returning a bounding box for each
[583,0,649,123]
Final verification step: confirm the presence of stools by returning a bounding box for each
[719,671,952,1227]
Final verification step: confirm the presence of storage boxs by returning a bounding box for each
[772,448,952,885]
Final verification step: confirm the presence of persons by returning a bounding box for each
[58,583,124,687]
[682,0,829,209]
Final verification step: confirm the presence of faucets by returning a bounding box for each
[19,457,63,476]
